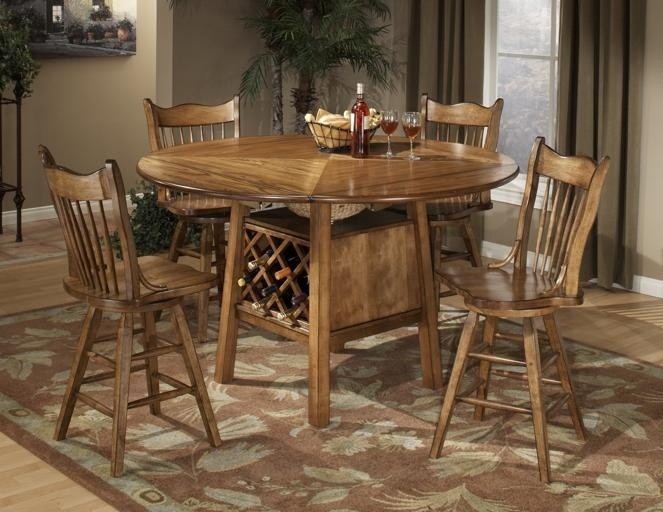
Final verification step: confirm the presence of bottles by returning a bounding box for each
[239,244,309,321]
[350,83,371,159]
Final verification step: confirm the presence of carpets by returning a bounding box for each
[1,297,662,512]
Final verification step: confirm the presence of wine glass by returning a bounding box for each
[379,110,422,159]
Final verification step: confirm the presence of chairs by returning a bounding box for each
[431,136,611,483]
[38,144,219,477]
[416,94,503,369]
[141,95,242,341]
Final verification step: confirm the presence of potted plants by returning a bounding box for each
[63,0,134,46]
